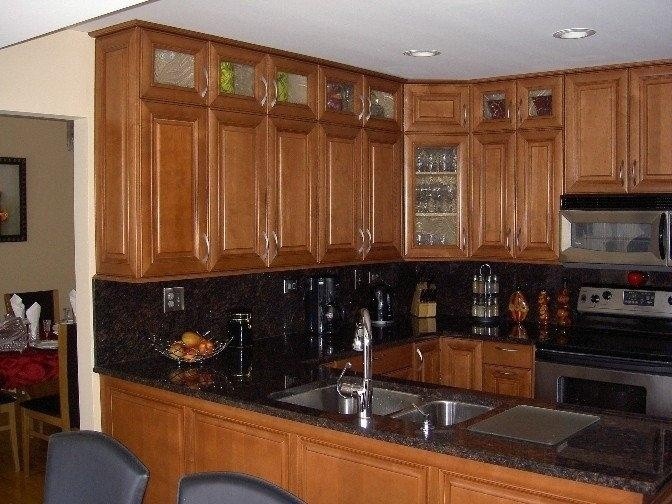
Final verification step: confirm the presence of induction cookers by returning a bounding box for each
[541,328,672,365]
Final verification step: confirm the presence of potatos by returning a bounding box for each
[168,331,215,362]
[170,367,214,391]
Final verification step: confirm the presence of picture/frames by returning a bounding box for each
[0,157,29,243]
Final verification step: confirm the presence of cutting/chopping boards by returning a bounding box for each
[468,403,601,447]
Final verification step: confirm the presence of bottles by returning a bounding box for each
[535,283,550,325]
[472,274,500,323]
[554,281,571,327]
[506,274,530,324]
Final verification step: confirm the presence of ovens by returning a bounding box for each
[536,353,672,422]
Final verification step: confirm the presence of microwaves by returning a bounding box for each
[559,204,672,267]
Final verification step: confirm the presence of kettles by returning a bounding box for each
[364,282,394,327]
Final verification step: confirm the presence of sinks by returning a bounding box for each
[393,401,494,428]
[268,377,423,419]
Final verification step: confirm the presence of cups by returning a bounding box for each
[414,150,458,248]
[27,323,39,343]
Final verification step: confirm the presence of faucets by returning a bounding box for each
[336,307,374,420]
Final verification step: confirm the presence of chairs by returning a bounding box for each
[43,429,150,504]
[176,471,306,504]
[0,289,79,482]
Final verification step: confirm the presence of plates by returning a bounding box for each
[29,340,59,350]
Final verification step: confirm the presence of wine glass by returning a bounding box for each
[43,320,51,340]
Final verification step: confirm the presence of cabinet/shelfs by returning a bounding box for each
[322,335,537,398]
